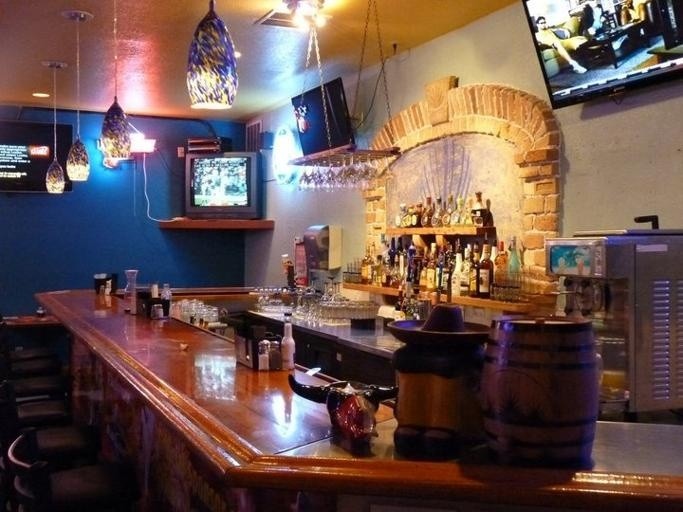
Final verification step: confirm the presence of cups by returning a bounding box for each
[319,300,381,319]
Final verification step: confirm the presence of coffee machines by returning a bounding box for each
[543,214,683,422]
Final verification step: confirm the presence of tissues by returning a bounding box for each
[375,305,400,334]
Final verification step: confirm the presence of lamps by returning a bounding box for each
[64,10,93,182]
[184,0,239,111]
[99,0,132,161]
[41,60,68,195]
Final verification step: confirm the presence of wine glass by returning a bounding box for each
[320,283,343,303]
[171,298,219,323]
[257,288,318,328]
[298,153,393,192]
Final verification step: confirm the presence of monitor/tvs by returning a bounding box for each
[185,152,263,219]
[291,77,356,157]
[0,119,74,194]
[521,0,683,110]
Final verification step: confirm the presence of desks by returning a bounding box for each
[1,314,63,350]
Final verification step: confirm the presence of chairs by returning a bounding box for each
[0,344,123,510]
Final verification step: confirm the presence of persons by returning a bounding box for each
[535,16,588,74]
[619,1,651,48]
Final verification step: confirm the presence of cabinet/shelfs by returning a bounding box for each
[344,224,536,316]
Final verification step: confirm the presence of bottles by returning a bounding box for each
[400,276,415,320]
[258,312,295,371]
[94,269,171,319]
[360,192,520,303]
